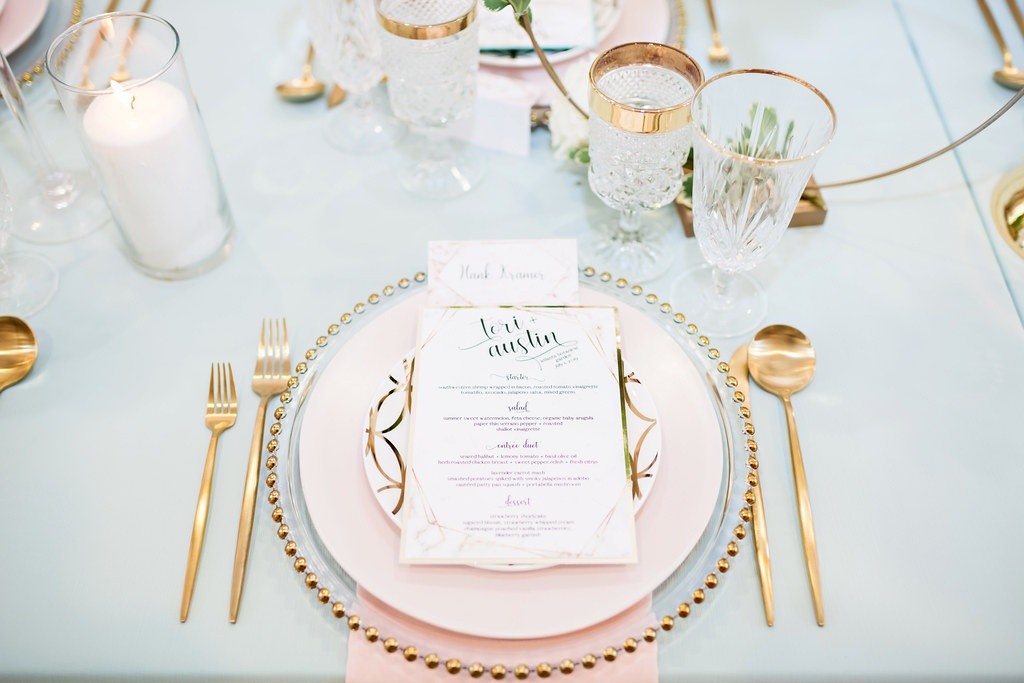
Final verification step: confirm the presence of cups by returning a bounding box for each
[45,10,240,281]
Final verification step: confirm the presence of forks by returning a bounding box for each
[180,363,238,621]
[230,317,292,624]
[705,1,730,64]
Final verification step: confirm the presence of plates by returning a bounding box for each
[265,262,759,679]
[479,1,621,66]
[0,0,47,56]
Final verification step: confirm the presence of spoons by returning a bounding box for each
[0,316,38,393]
[274,45,325,102]
[746,323,827,627]
[976,0,1024,91]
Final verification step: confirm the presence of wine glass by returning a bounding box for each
[301,0,407,156]
[587,41,706,283]
[0,50,112,240]
[375,1,489,202]
[671,67,837,339]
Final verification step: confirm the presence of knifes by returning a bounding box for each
[723,340,776,629]
[327,83,346,107]
